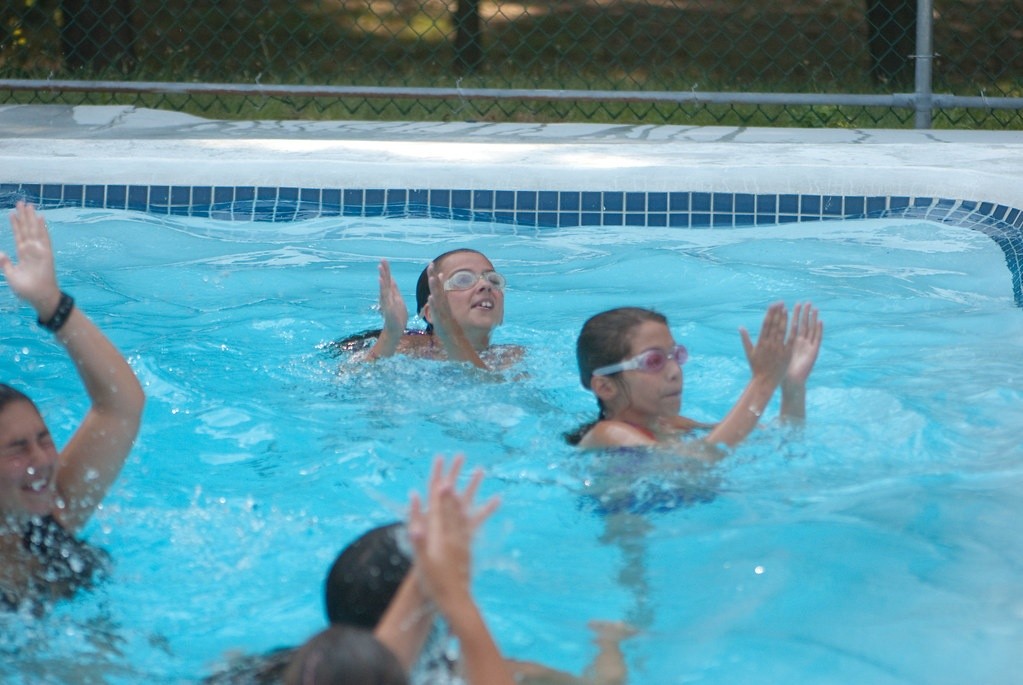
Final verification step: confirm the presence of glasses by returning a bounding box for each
[416,271,505,319]
[592,346,688,377]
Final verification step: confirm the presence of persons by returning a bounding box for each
[0,201,146,605]
[573,301,823,448]
[367,248,505,366]
[275,455,513,685]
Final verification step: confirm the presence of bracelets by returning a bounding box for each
[38,292,72,331]
